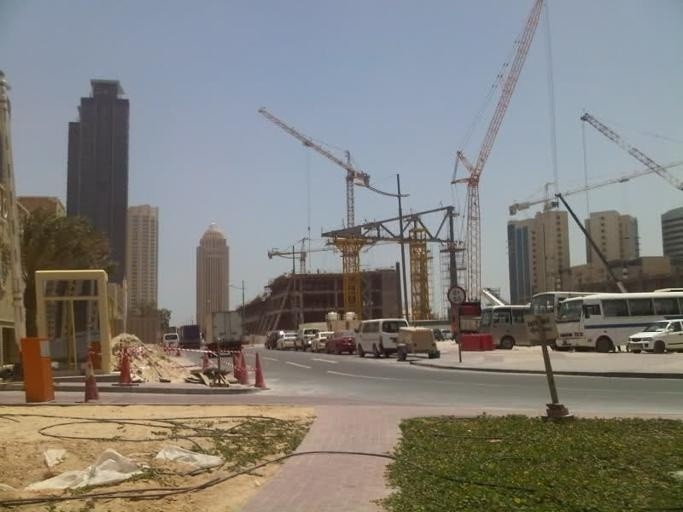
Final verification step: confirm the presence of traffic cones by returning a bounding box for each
[112,347,140,386]
[83,355,103,403]
[252,352,268,387]
[161,343,251,386]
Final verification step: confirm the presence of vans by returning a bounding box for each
[353,318,411,358]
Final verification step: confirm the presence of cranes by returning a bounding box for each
[266,237,342,273]
[506,113,682,214]
[257,106,371,232]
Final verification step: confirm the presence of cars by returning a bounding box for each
[439,329,454,339]
[264,327,355,355]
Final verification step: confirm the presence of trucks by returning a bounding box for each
[161,311,244,350]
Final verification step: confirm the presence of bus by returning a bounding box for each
[476,288,683,353]
